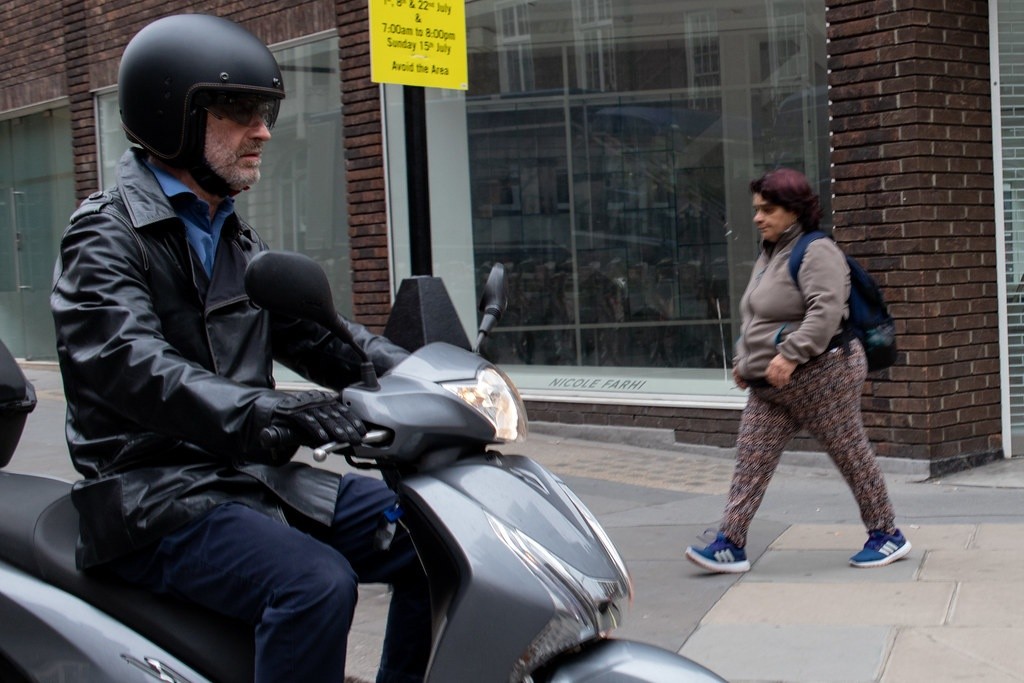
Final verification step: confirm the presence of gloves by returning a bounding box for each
[252,388,367,447]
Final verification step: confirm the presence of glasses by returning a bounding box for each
[754,202,776,216]
[208,91,281,130]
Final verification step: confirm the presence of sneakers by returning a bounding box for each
[848,528,912,567]
[683,528,751,573]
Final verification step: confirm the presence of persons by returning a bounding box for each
[685,168,912,573]
[49,14,435,683]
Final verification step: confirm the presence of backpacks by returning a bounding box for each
[787,232,898,373]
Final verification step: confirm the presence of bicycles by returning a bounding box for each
[480,256,730,366]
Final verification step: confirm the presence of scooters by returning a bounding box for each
[0,249,728,682]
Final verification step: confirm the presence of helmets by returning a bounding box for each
[118,12,285,162]
